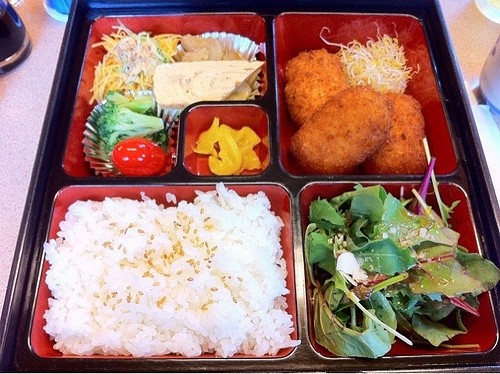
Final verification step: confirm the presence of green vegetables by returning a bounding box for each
[305,136,500,359]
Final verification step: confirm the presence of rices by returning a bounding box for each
[38,183,302,358]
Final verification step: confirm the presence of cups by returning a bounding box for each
[0,1,34,75]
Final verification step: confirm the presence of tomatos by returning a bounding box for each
[111,137,168,177]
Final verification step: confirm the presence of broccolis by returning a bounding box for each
[96,90,169,156]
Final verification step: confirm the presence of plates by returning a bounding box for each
[180,101,272,179]
[59,10,269,180]
[295,175,500,365]
[271,9,459,182]
[27,182,302,363]
